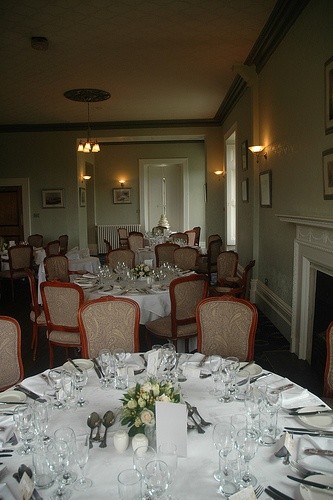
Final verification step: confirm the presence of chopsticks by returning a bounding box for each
[233,372,270,386]
[0,449,12,457]
[283,426,333,438]
[13,385,47,404]
[265,486,295,500]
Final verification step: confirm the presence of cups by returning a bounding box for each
[117,469,142,500]
[10,240,15,245]
[217,445,241,497]
[159,445,177,482]
[258,408,278,446]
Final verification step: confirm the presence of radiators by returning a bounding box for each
[96,224,142,254]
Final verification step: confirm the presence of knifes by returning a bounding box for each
[68,357,83,374]
[289,410,333,415]
[286,475,333,491]
[91,360,101,379]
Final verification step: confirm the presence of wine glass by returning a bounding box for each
[150,235,187,249]
[13,344,282,500]
[98,260,175,290]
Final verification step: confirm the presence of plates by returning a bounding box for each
[299,473,333,500]
[237,364,262,376]
[296,407,333,430]
[0,391,27,411]
[63,359,94,372]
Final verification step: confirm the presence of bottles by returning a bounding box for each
[111,429,129,453]
[131,432,148,453]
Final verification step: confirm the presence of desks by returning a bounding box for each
[37,256,101,306]
[75,268,197,326]
[0,352,333,500]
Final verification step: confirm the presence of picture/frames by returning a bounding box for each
[79,187,87,207]
[259,169,272,208]
[324,55,333,135]
[112,187,131,204]
[322,148,333,200]
[41,188,65,208]
[241,139,248,202]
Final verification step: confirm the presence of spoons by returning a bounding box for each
[99,410,114,448]
[86,412,100,449]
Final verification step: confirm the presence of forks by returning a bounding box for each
[254,485,264,498]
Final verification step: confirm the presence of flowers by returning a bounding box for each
[117,371,185,438]
[130,263,152,278]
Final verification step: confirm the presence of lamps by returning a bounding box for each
[63,88,112,152]
[81,175,92,183]
[119,180,125,187]
[247,145,268,163]
[214,171,223,180]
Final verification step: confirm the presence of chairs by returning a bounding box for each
[0,226,258,392]
